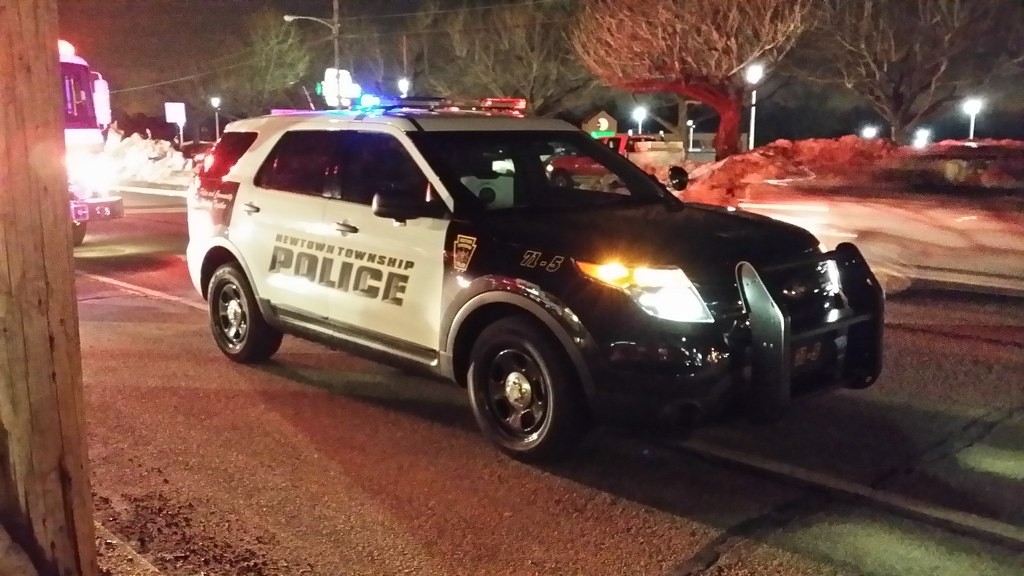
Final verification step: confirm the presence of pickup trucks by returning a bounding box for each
[549,134,685,190]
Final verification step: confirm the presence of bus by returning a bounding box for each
[54,44,126,246]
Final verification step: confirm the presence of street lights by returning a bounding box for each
[746,64,765,151]
[965,100,981,140]
[282,12,345,111]
[631,103,649,132]
[203,97,225,145]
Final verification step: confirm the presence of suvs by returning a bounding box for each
[184,94,891,459]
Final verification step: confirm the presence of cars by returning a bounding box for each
[687,139,707,148]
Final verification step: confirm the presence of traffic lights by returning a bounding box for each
[312,82,323,96]
[360,93,379,109]
